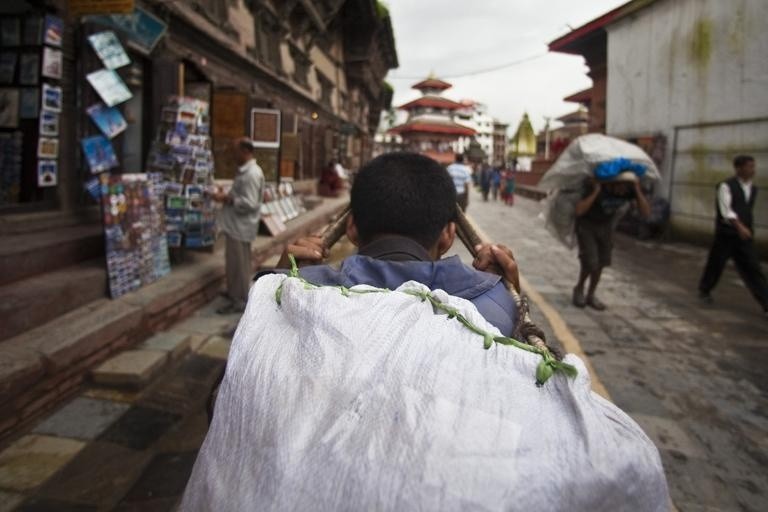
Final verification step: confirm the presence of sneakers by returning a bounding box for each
[572,285,586,308]
[586,294,607,311]
[216,302,244,314]
[219,289,233,300]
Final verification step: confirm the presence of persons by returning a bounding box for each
[211,136,265,315]
[572,169,650,311]
[204,150,520,429]
[445,153,472,213]
[318,160,344,197]
[473,160,514,207]
[697,155,768,314]
